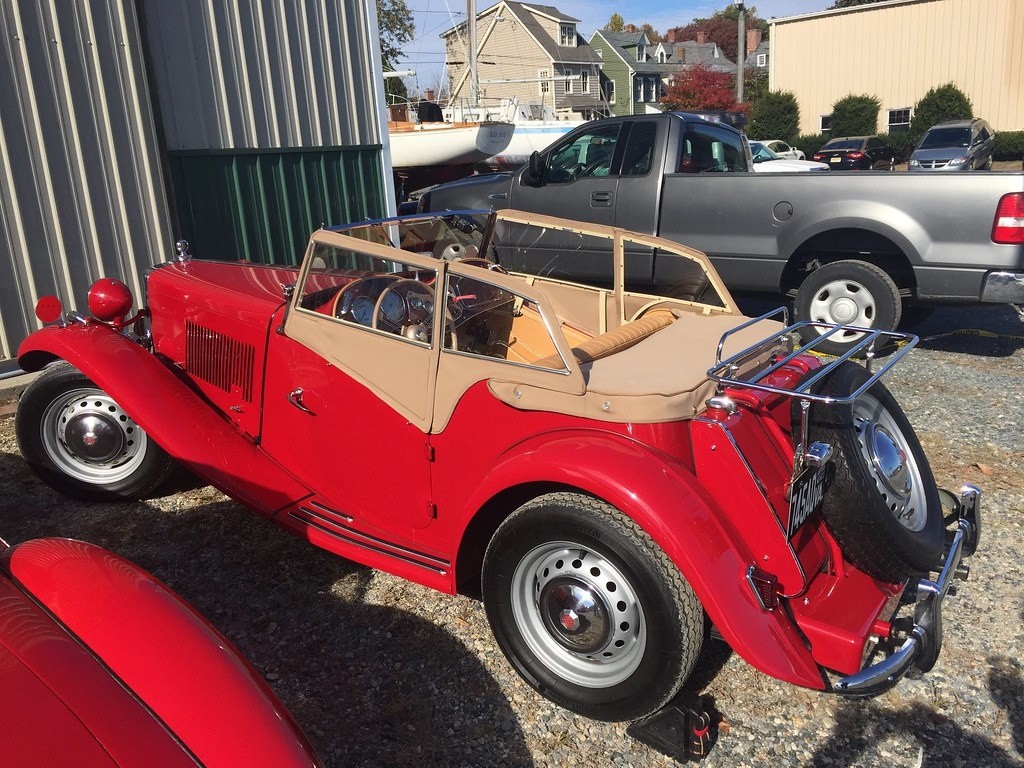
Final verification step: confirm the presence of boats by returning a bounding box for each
[376,1,518,216]
[443,0,619,166]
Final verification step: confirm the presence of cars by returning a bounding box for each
[14,208,982,723]
[813,135,896,172]
[751,139,806,160]
[747,140,832,174]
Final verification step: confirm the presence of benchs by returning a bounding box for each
[527,310,677,369]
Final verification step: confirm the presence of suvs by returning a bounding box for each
[908,116,998,172]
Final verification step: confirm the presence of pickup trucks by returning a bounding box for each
[416,111,1023,358]
